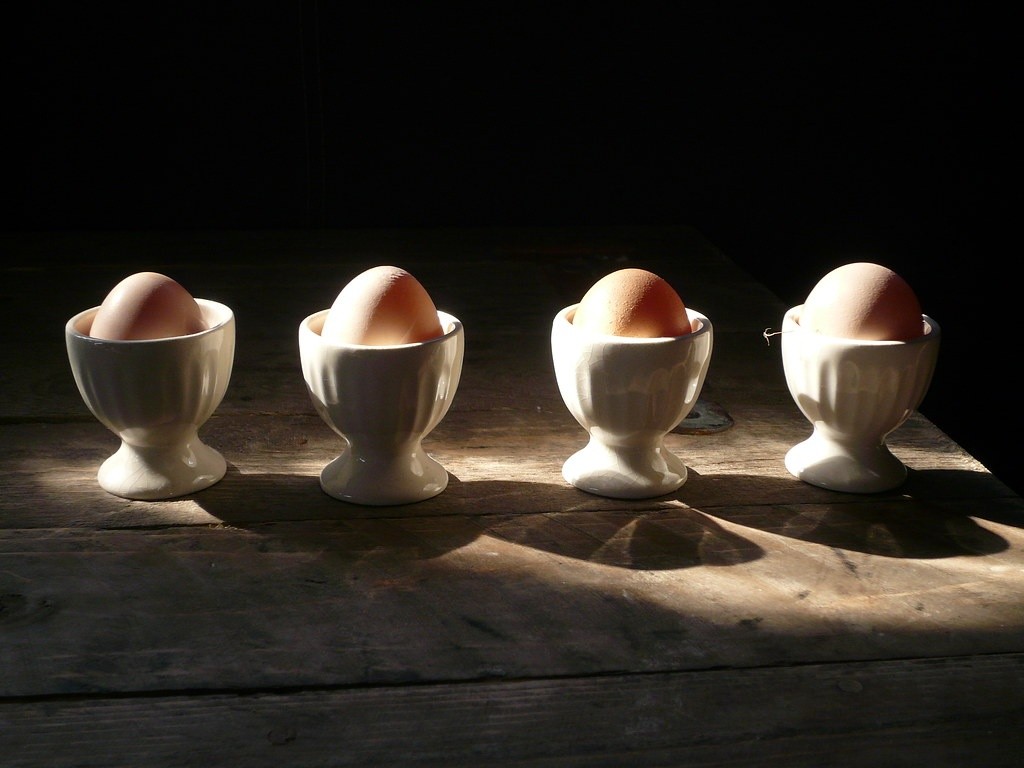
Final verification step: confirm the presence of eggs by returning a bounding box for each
[571,269,691,339]
[799,262,925,341]
[89,271,210,340]
[320,265,444,346]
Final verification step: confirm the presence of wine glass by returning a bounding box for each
[66,299,235,499]
[782,306,938,494]
[299,309,464,505]
[551,303,712,497]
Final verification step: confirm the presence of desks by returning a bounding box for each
[0,224,1024,768]
